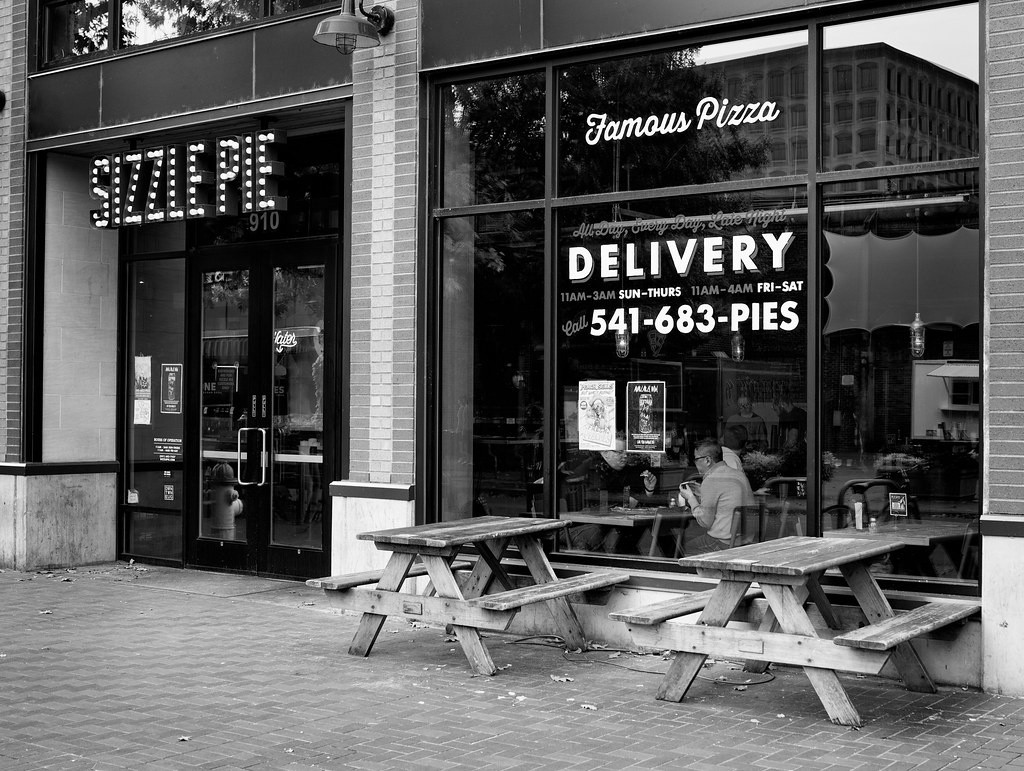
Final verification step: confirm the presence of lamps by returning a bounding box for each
[310,1,396,55]
[613,235,629,359]
[730,326,744,363]
[909,216,926,358]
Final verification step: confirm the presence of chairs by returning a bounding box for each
[557,490,982,580]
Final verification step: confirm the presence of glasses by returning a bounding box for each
[693,456,706,463]
[611,450,623,455]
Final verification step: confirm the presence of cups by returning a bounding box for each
[779,483,788,502]
[623,487,629,509]
[309,446,318,456]
[600,490,608,515]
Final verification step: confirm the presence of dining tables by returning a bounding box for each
[822,516,981,547]
[558,505,697,528]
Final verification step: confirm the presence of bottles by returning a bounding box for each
[868,518,878,533]
[670,497,676,508]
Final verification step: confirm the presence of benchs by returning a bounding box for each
[305,558,471,590]
[832,599,980,653]
[465,572,632,611]
[608,586,768,626]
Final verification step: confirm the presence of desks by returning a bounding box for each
[346,513,587,674]
[653,531,939,729]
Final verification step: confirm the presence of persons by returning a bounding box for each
[552,396,806,560]
